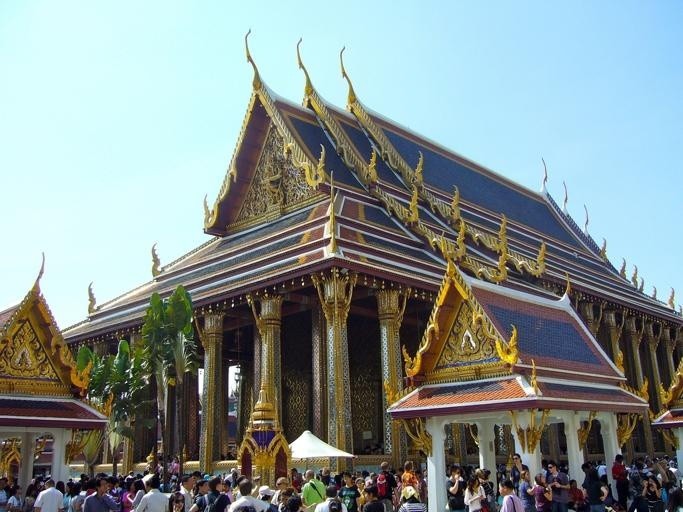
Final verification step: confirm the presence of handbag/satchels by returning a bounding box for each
[480,488,490,512]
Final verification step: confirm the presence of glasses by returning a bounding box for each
[548,467,553,469]
[513,457,518,460]
[174,499,184,504]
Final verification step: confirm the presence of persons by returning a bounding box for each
[0,456,428,512]
[446,455,683,512]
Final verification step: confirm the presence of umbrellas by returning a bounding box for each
[287,430,355,471]
